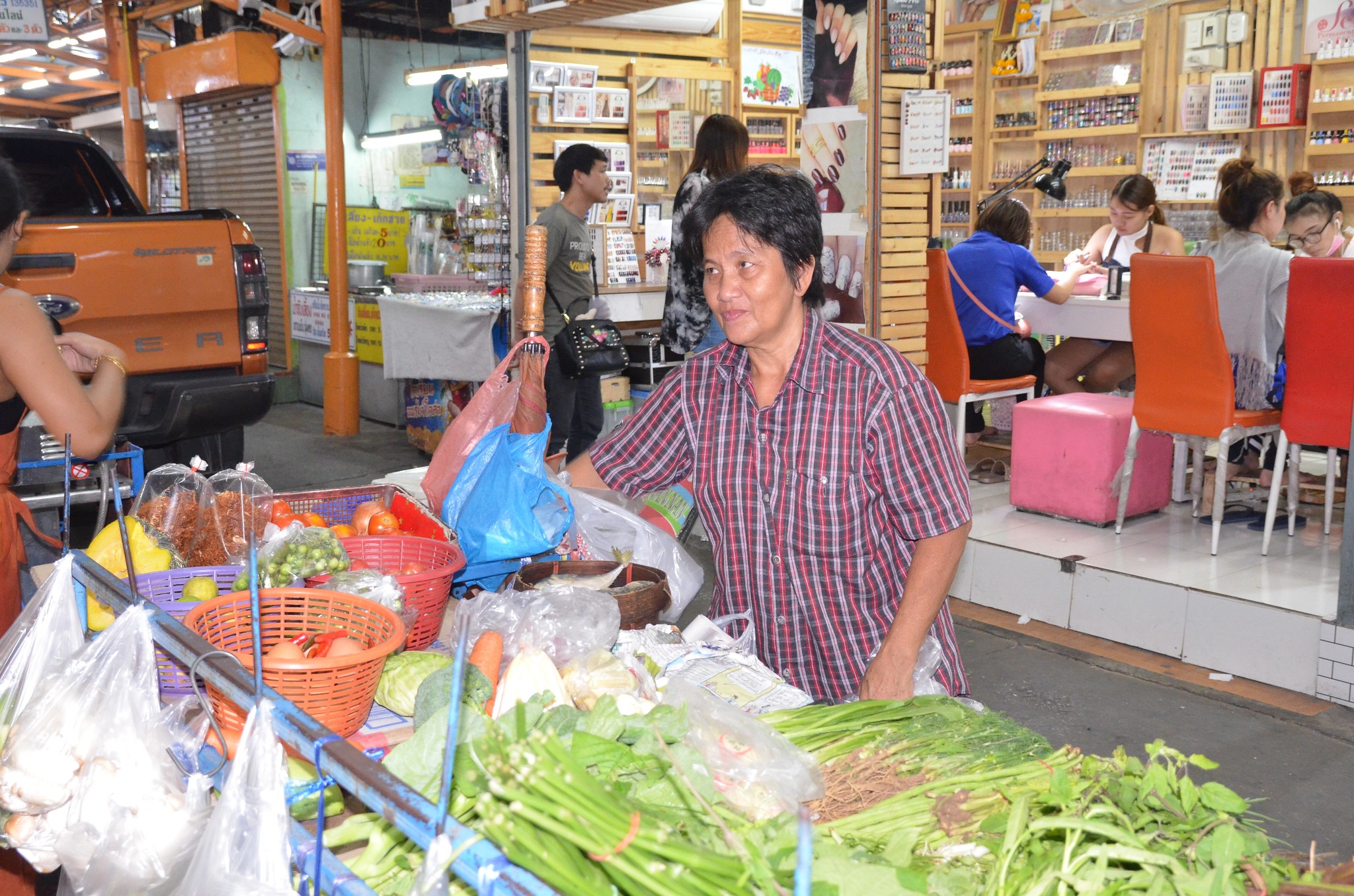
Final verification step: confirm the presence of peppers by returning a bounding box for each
[286,631,376,659]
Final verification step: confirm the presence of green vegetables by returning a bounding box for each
[798,715,1354,896]
[312,698,797,896]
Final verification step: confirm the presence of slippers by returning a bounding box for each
[1247,512,1306,531]
[968,458,996,479]
[1200,504,1263,525]
[979,460,1010,484]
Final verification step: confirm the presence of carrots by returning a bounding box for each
[200,728,365,761]
[467,630,503,718]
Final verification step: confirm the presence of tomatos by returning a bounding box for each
[257,500,411,536]
[351,557,443,648]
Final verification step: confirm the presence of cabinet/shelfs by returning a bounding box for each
[638,110,695,197]
[941,0,1354,272]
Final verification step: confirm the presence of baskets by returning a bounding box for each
[180,587,406,739]
[96,564,306,696]
[304,535,467,652]
[249,483,460,547]
[393,273,487,294]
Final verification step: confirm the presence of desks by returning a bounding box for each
[1012,292,1261,502]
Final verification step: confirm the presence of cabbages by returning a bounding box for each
[373,648,455,716]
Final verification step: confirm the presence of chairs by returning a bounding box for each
[1115,253,1301,555]
[1262,257,1354,556]
[895,249,1037,458]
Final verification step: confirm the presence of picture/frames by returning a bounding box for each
[563,63,598,87]
[593,87,630,124]
[553,86,593,124]
[528,61,564,93]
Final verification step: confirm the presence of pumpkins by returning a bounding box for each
[83,515,185,632]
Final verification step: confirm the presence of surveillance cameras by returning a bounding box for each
[271,33,304,59]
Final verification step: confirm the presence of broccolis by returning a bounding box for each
[412,660,494,731]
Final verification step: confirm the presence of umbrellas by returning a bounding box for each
[508,226,549,439]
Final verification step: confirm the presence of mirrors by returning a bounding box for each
[628,63,734,221]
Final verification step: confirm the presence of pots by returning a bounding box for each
[346,259,388,289]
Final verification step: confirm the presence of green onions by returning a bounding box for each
[756,692,946,762]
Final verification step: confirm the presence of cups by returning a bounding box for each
[408,214,462,275]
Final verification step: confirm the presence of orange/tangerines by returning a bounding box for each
[154,577,249,685]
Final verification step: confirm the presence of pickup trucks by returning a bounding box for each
[0,118,276,479]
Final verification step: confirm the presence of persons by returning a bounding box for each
[560,164,973,703]
[1043,173,1186,397]
[1283,172,1354,258]
[660,114,749,356]
[805,0,858,109]
[1189,155,1318,486]
[510,143,612,465]
[0,156,126,639]
[945,195,1097,444]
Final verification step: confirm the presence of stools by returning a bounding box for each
[1009,392,1174,529]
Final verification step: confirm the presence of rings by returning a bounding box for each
[58,346,61,350]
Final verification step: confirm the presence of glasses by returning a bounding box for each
[1288,213,1336,249]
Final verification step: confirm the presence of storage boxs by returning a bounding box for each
[391,273,488,294]
[596,390,649,441]
[620,331,686,392]
[599,376,631,404]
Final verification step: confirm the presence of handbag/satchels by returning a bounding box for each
[421,335,550,517]
[0,552,86,757]
[1012,317,1030,337]
[711,608,757,657]
[0,600,299,896]
[441,412,574,592]
[553,319,629,379]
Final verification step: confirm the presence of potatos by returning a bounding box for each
[264,637,364,658]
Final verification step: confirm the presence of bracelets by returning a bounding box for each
[95,355,126,377]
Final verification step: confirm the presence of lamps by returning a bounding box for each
[403,0,508,86]
[976,150,1071,215]
[358,26,442,150]
